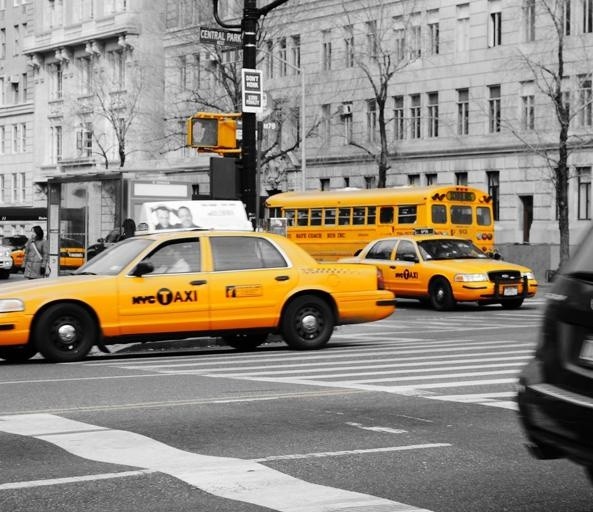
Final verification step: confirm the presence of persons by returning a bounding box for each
[149,205,176,231]
[149,244,192,273]
[20,225,50,282]
[173,206,200,230]
[118,217,136,242]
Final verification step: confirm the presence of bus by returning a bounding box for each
[263,184,494,262]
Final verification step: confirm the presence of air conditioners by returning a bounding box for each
[337,104,352,116]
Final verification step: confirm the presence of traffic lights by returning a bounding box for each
[187,112,237,149]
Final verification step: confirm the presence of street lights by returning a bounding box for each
[245,44,306,191]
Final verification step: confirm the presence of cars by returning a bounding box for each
[9,235,85,274]
[0,229,398,364]
[0,243,14,280]
[335,228,538,311]
[517,225,593,484]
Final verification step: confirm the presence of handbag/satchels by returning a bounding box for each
[44,261,51,276]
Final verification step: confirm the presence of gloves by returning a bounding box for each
[40,267,46,275]
[22,267,25,273]
